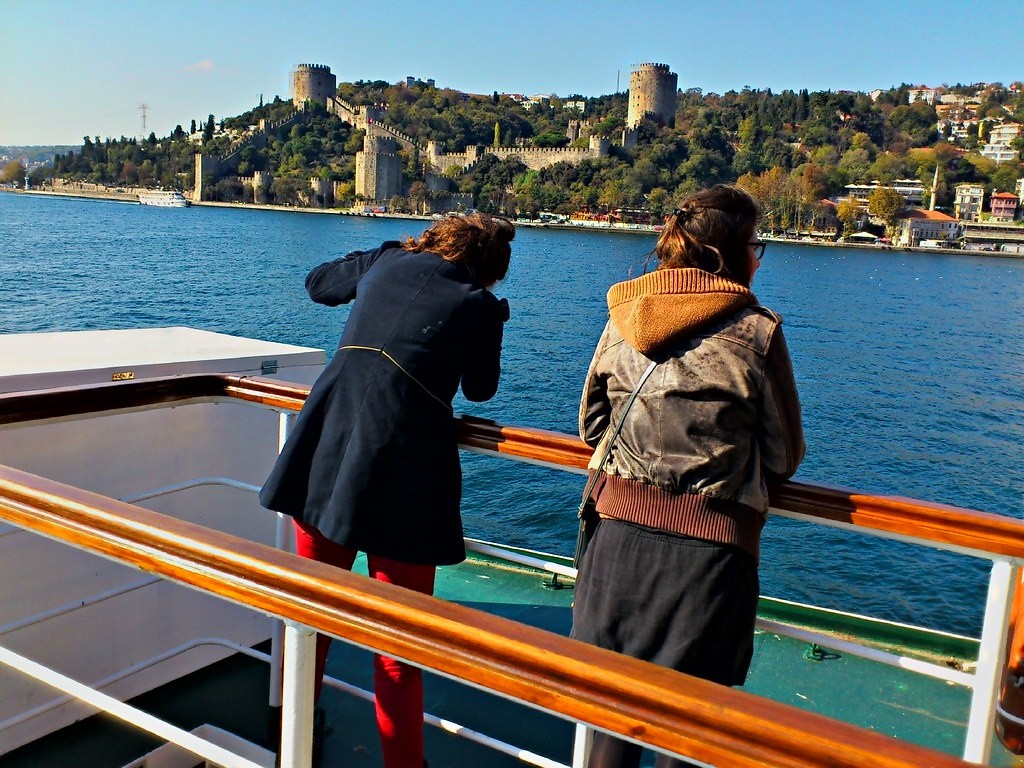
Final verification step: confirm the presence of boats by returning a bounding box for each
[137,190,189,207]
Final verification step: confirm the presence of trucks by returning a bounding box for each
[920,241,941,248]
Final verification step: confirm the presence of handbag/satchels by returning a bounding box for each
[573,502,602,570]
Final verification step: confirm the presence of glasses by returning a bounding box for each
[747,242,766,260]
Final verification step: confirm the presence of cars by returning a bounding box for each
[777,234,813,242]
[963,243,992,251]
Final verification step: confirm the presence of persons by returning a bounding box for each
[574,184,805,768]
[264,209,515,768]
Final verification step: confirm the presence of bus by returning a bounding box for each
[928,240,949,248]
